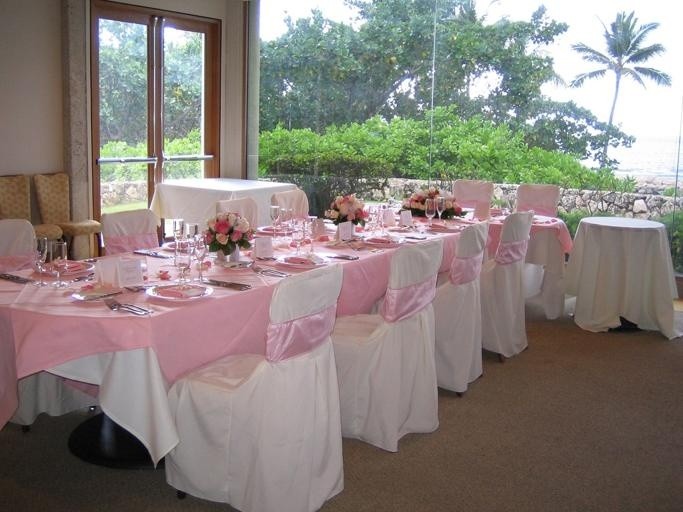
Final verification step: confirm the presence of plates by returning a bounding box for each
[71,290,113,304]
[363,237,405,247]
[164,241,207,250]
[146,284,213,302]
[45,259,95,275]
[258,226,298,234]
[277,256,329,268]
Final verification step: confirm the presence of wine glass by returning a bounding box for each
[173,220,206,283]
[425,199,446,227]
[49,242,68,290]
[270,206,321,258]
[369,206,381,228]
[33,237,49,287]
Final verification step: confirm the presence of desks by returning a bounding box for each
[561,217,683,340]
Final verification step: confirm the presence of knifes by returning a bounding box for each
[73,291,122,304]
[203,278,252,291]
